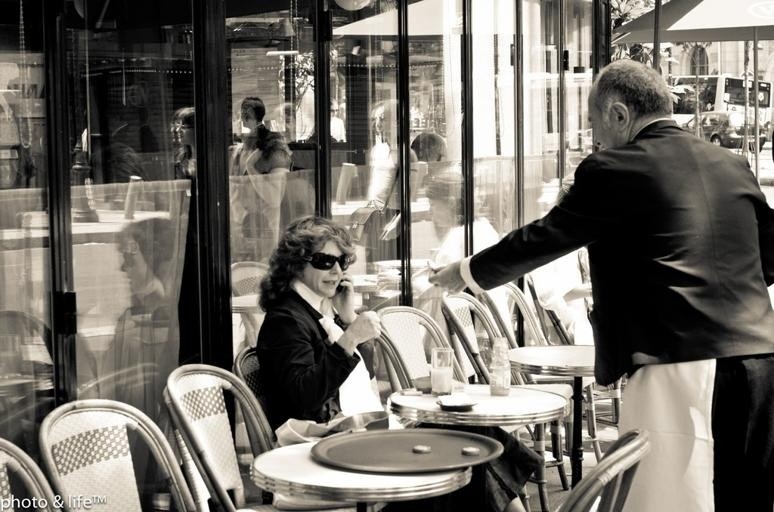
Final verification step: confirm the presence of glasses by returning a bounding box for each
[303,251,350,270]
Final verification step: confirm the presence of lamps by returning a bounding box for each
[275,16,296,38]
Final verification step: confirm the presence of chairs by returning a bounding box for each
[555,427,652,512]
[442,290,524,384]
[237,346,262,381]
[232,261,271,343]
[39,399,196,512]
[480,282,546,349]
[526,275,622,424]
[375,305,468,391]
[0,438,63,512]
[76,360,164,423]
[164,364,277,512]
[0,310,56,375]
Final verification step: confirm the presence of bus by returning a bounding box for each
[665,74,773,142]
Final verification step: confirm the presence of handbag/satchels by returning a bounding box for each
[271,411,389,509]
[346,202,382,248]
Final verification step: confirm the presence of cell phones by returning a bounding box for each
[332,270,350,295]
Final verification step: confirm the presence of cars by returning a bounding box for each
[682,111,767,154]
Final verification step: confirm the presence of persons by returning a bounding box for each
[427,56,773,512]
[113,89,499,265]
[96,218,182,493]
[257,215,543,512]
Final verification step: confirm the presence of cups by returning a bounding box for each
[429,346,453,396]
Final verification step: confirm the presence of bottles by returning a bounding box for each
[488,336,511,396]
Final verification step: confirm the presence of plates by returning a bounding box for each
[434,394,479,410]
